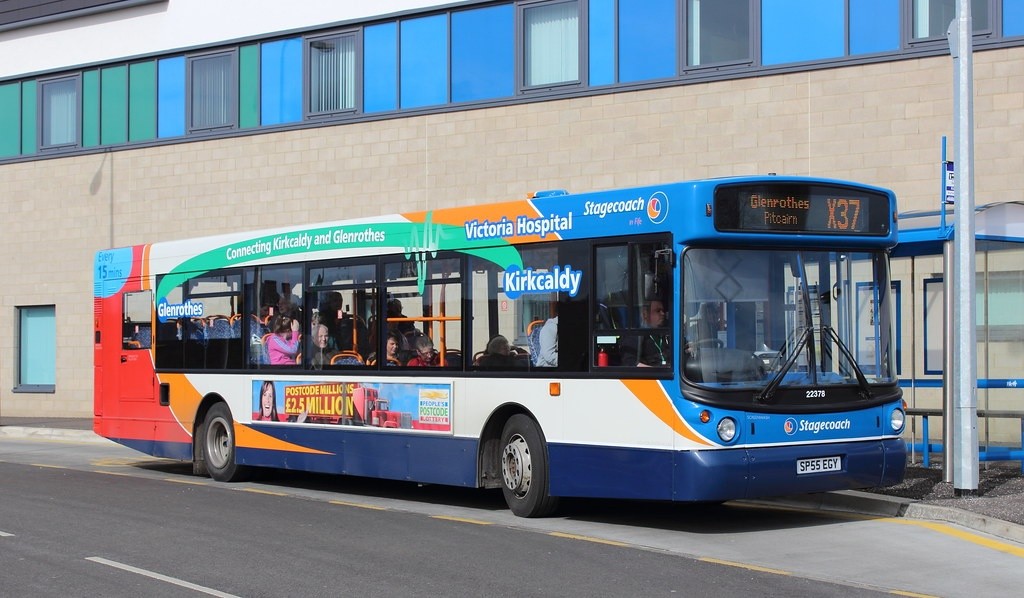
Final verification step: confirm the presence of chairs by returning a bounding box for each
[128,314,549,372]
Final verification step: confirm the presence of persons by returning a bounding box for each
[620,298,695,367]
[251,283,532,366]
[686,302,724,348]
[256,380,280,422]
[535,301,558,367]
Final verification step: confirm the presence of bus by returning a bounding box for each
[91,170,909,518]
